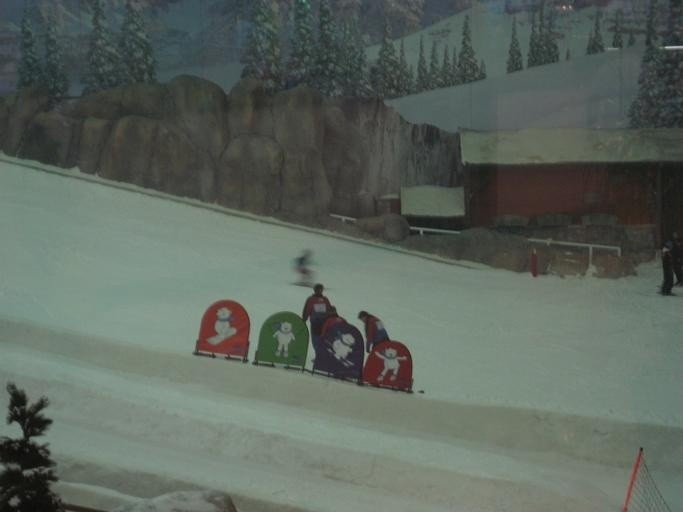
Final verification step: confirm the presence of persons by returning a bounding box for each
[300,282,331,362]
[291,247,320,284]
[658,241,676,295]
[318,304,350,341]
[670,228,682,286]
[357,310,390,353]
